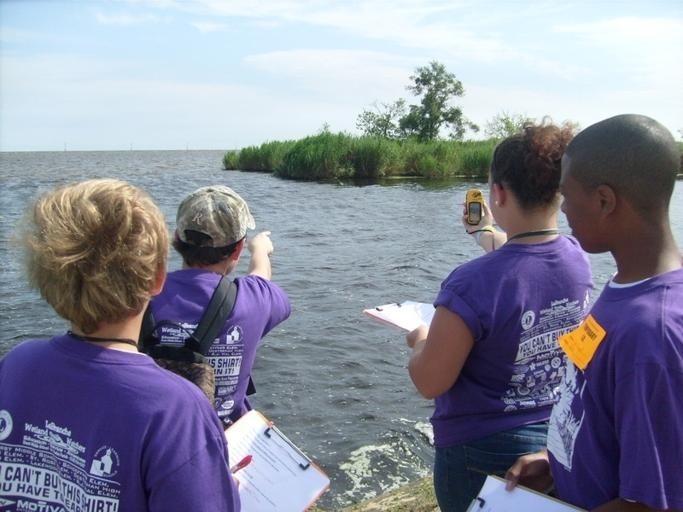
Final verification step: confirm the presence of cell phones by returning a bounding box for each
[467,189,483,224]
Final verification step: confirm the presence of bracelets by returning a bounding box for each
[476,225,493,244]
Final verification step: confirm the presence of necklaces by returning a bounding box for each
[65,330,140,350]
[507,228,560,243]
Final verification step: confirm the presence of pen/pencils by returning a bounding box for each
[230,454,252,474]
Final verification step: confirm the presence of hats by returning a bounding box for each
[176,185,256,248]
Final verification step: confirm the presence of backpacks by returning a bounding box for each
[143,271,238,410]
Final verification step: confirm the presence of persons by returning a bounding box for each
[403,114,600,512]
[125,183,293,426]
[0,174,245,511]
[495,113,683,512]
